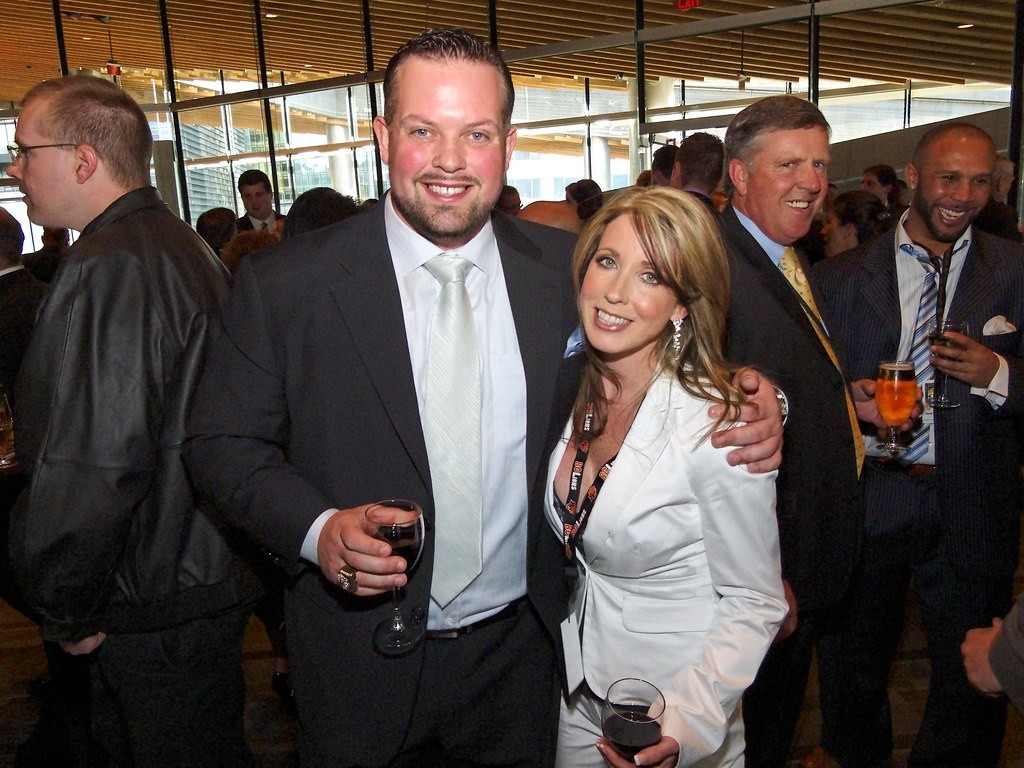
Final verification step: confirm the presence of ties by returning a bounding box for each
[779,245,865,482]
[261,222,269,232]
[895,240,969,469]
[421,252,482,610]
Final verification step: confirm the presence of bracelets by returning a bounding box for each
[774,388,789,418]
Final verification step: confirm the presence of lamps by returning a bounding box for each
[106,30,121,76]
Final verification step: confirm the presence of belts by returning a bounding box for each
[872,456,935,476]
[426,594,529,639]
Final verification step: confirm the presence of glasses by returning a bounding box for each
[6,143,102,167]
[499,204,523,211]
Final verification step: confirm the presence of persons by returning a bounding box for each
[960,587,1024,716]
[715,95,921,768]
[811,123,1024,768]
[542,185,791,768]
[1,28,1024,768]
[1,76,252,768]
[184,29,785,768]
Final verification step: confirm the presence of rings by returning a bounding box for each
[337,565,359,593]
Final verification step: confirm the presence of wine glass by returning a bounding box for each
[0,394,18,469]
[928,320,969,408]
[875,361,916,451]
[365,500,425,647]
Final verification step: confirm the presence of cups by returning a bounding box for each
[602,678,666,763]
